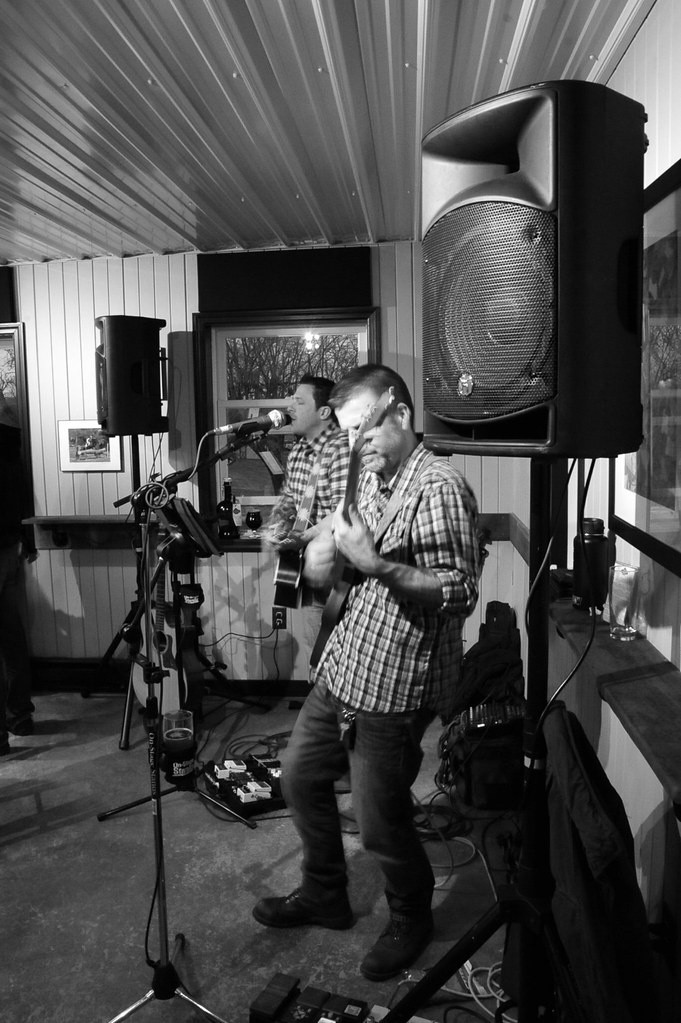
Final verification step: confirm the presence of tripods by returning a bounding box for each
[382,457,583,1023]
[83,425,261,1023]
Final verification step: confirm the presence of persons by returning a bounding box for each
[254,364,482,981]
[0,423,35,757]
[262,373,350,686]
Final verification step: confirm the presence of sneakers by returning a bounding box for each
[252,877,353,930]
[360,909,434,982]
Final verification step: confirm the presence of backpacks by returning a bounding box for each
[439,599,527,811]
[494,697,654,1023]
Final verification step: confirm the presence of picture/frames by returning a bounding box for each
[58,420,123,472]
[607,157,681,581]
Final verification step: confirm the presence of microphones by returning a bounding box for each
[206,410,288,437]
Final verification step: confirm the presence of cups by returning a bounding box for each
[162,710,194,745]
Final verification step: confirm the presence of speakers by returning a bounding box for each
[413,79,647,460]
[94,315,169,438]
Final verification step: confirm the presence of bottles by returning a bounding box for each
[217,478,240,539]
[609,565,640,641]
[573,517,608,609]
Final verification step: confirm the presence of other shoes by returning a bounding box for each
[6,717,33,735]
[0,744,10,756]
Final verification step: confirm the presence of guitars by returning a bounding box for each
[309,386,396,669]
[131,520,204,717]
[268,528,303,610]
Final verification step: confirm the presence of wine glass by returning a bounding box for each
[246,510,263,538]
